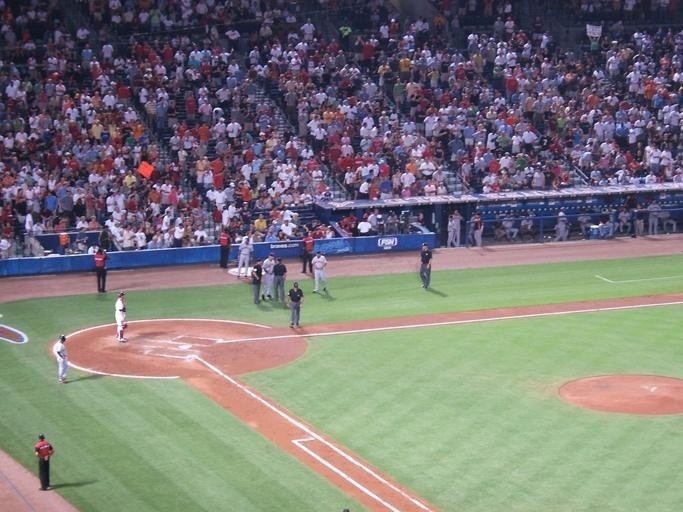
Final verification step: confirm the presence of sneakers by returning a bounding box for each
[58,378,65,383]
[313,290,319,293]
[261,294,264,300]
[267,295,272,301]
[39,487,47,491]
[323,287,327,291]
[119,336,127,342]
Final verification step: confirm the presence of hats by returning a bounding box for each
[255,258,261,262]
[294,283,298,287]
[270,253,275,256]
[278,258,281,261]
[422,243,427,246]
[59,335,65,340]
[118,293,124,297]
[316,251,320,254]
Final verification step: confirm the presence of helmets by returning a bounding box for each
[39,434,44,439]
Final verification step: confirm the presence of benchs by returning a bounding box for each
[349,2,640,200]
[517,219,662,235]
[105,29,349,244]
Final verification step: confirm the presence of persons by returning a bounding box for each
[263,256,288,306]
[261,251,275,303]
[2,0,683,258]
[309,249,329,294]
[92,246,111,294]
[31,432,55,493]
[303,232,315,272]
[112,291,130,343]
[219,227,254,278]
[250,257,263,305]
[287,281,304,330]
[50,334,70,385]
[417,242,433,290]
